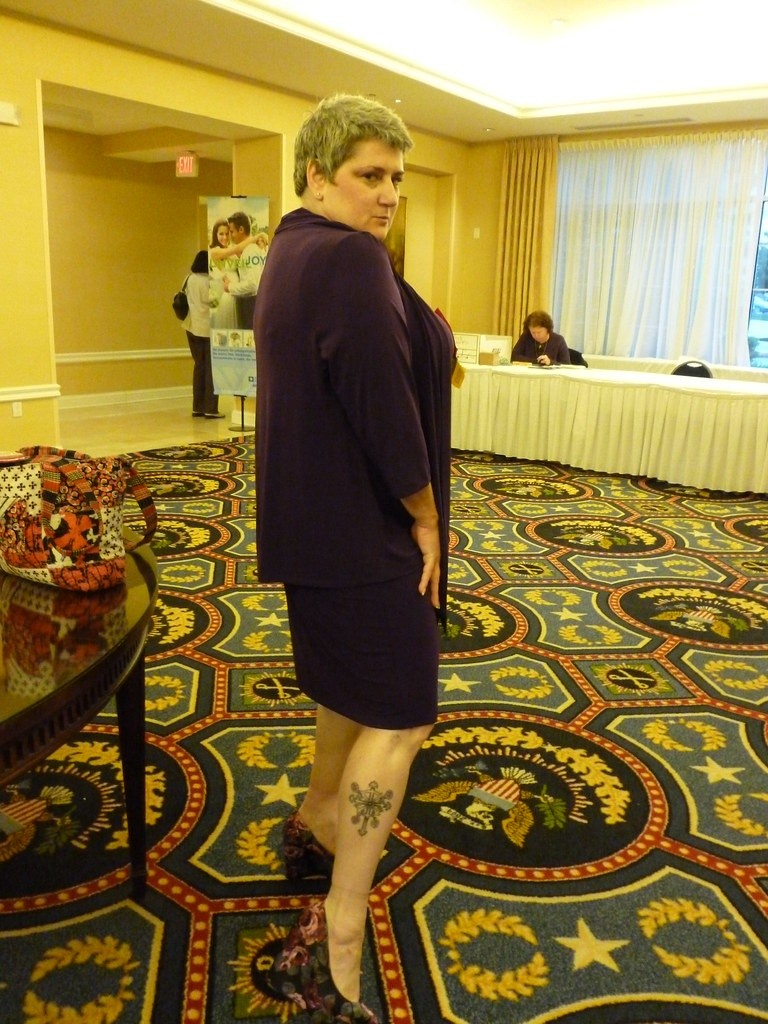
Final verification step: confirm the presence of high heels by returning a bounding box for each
[277,810,383,1024]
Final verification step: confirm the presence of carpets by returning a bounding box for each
[0,431,768,1023]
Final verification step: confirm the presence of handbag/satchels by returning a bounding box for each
[0,446,157,594]
[171,292,188,321]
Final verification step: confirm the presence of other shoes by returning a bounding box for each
[192,411,226,419]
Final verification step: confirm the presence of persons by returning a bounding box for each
[253,94,459,1023]
[209,218,268,329]
[183,250,225,419]
[510,311,571,366]
[222,212,267,329]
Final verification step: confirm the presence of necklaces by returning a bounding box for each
[534,339,547,357]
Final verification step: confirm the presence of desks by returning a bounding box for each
[0,519,160,905]
[448,362,768,500]
